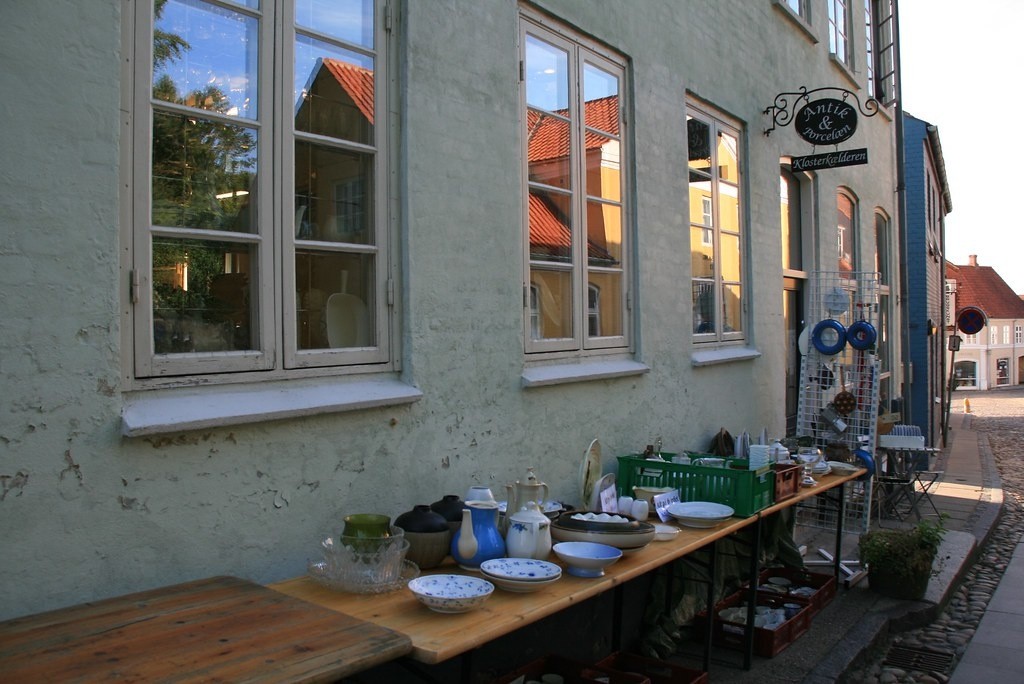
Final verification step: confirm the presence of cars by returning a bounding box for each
[968,373,975,378]
[201,272,309,350]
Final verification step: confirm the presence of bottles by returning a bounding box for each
[394,487,496,571]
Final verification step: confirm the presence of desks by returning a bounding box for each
[756,459,877,589]
[878,443,942,525]
[0,577,415,684]
[254,507,757,684]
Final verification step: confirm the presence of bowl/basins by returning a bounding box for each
[825,461,860,476]
[309,525,410,584]
[552,541,623,577]
[408,574,495,613]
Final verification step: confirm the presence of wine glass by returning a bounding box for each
[797,447,821,485]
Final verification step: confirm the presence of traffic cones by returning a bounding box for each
[964,398,970,413]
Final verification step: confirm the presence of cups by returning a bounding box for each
[718,603,803,630]
[749,445,770,482]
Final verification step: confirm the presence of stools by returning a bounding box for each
[873,472,906,522]
[904,468,945,516]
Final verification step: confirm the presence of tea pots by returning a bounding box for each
[502,466,552,561]
[451,501,505,571]
[769,439,790,461]
[692,458,733,468]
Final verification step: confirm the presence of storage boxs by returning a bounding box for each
[878,434,925,448]
[594,649,711,684]
[695,589,812,657]
[772,463,801,503]
[616,450,777,517]
[745,564,838,616]
[495,650,652,684]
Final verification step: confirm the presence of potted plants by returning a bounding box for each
[859,510,955,600]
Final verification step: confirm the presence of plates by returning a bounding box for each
[667,501,735,528]
[305,558,420,594]
[717,427,768,459]
[652,522,680,541]
[761,575,816,596]
[497,499,563,521]
[480,558,562,593]
[578,438,602,511]
[811,460,832,477]
